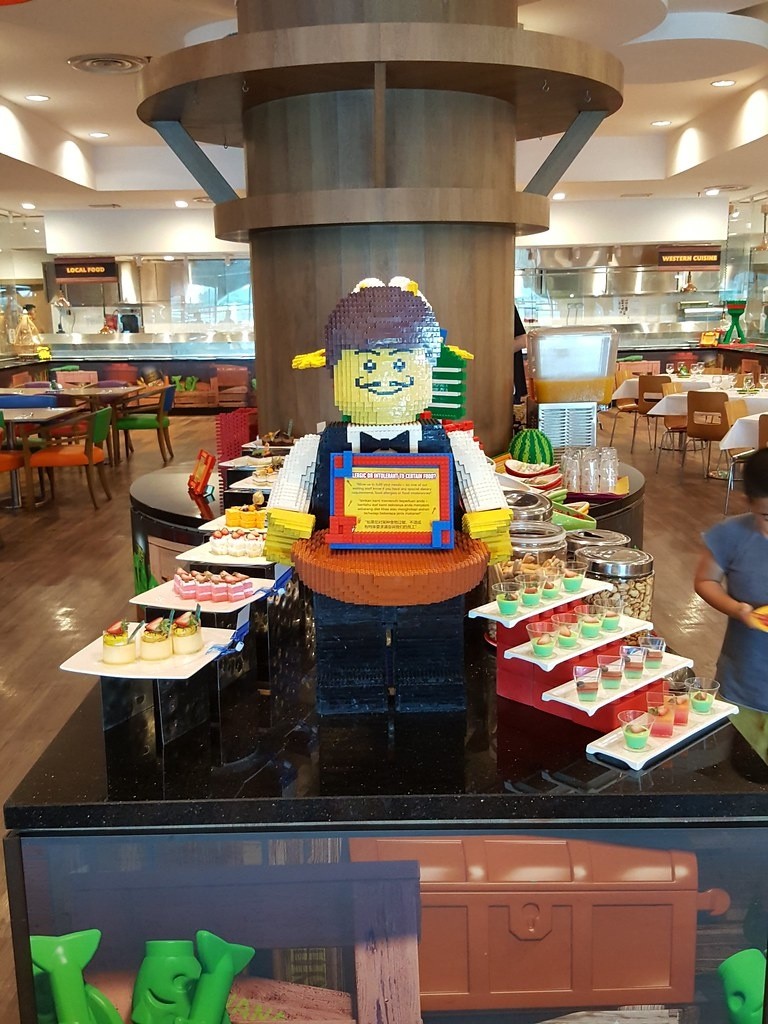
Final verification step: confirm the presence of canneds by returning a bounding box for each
[483,493,656,647]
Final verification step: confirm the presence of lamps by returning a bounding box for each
[753,232,768,251]
[224,255,231,267]
[530,248,538,260]
[614,245,621,258]
[682,271,697,292]
[54,284,70,307]
[23,222,27,229]
[136,256,142,267]
[7,214,14,224]
[574,248,580,259]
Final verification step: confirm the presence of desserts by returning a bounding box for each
[491,560,719,750]
[209,528,267,558]
[102,603,203,665]
[173,567,254,603]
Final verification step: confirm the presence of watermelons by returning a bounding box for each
[508,429,554,466]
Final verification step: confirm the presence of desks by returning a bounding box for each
[718,411,768,450]
[612,374,738,451]
[0,387,146,464]
[647,387,768,480]
[0,407,83,509]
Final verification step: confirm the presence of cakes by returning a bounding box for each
[252,454,284,486]
[247,442,276,464]
[261,430,294,446]
[224,491,269,530]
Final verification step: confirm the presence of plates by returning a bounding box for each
[505,460,560,475]
[220,456,284,467]
[128,577,275,613]
[229,476,272,489]
[176,542,273,566]
[522,474,563,490]
[198,515,268,533]
[241,439,299,449]
[59,621,239,679]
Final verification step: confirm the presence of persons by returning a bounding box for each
[513,305,527,405]
[694,447,768,761]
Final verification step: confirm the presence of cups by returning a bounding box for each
[562,446,619,493]
[572,666,602,702]
[553,613,584,651]
[557,562,588,594]
[618,711,657,754]
[514,574,547,608]
[645,691,676,738]
[536,567,565,600]
[491,582,526,618]
[619,645,648,678]
[594,599,626,633]
[638,637,666,669]
[684,677,720,715]
[526,622,560,660]
[678,362,685,371]
[597,655,626,690]
[574,605,607,640]
[662,681,691,726]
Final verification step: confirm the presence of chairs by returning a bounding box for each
[682,391,729,480]
[75,380,134,464]
[35,394,98,474]
[0,410,30,508]
[759,414,768,448]
[656,382,706,479]
[723,399,757,515]
[702,353,763,388]
[57,371,98,411]
[609,370,652,453]
[0,381,55,443]
[111,384,178,466]
[630,376,676,457]
[22,405,112,512]
[0,396,57,499]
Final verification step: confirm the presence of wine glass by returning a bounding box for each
[690,364,698,379]
[759,374,768,391]
[697,362,705,379]
[728,372,738,391]
[666,364,674,376]
[744,376,754,395]
[712,376,723,391]
